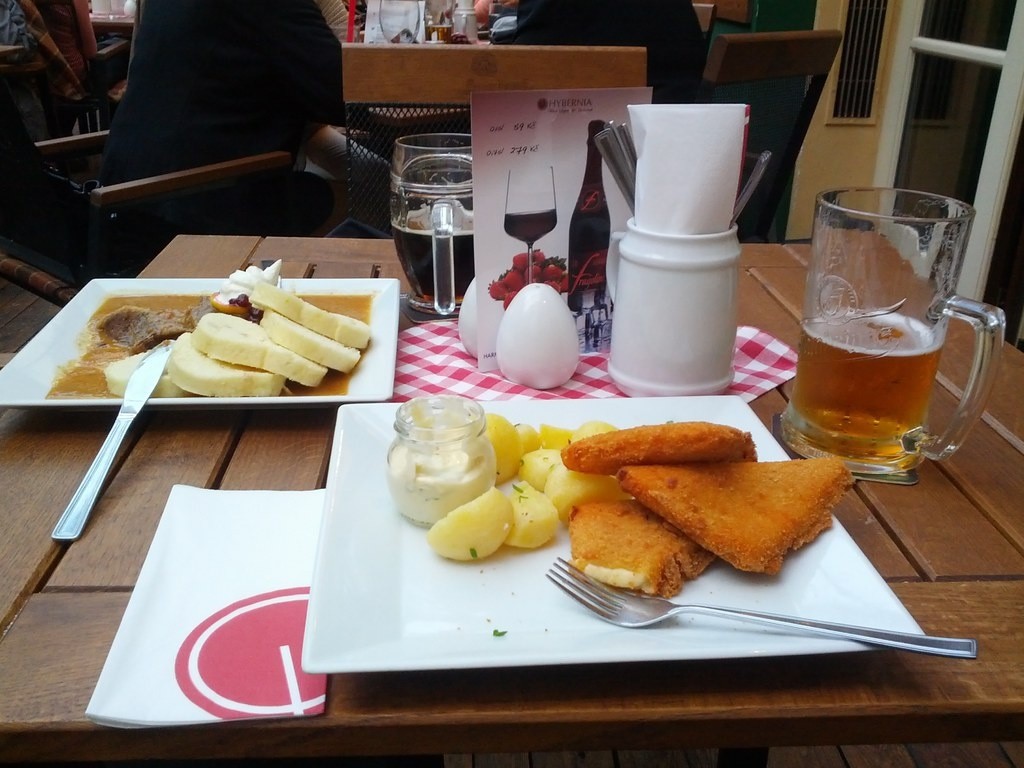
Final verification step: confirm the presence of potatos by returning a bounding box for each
[423,412,633,563]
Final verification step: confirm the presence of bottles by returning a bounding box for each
[566,119,611,313]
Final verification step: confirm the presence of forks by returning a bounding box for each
[543,554,980,663]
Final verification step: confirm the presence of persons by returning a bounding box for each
[31,0,130,137]
[97,0,395,272]
[473,0,709,105]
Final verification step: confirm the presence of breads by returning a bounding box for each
[104,282,372,399]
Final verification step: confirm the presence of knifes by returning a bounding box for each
[50,343,174,543]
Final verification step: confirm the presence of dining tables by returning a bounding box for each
[0,233,1024,768]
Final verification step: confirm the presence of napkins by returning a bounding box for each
[85,484,329,731]
[627,102,750,236]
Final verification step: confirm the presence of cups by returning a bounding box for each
[779,187,1010,488]
[388,132,478,316]
[605,218,743,395]
[90,0,126,16]
[487,3,502,30]
[453,8,477,43]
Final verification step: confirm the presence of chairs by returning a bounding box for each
[698,27,843,244]
[338,39,650,239]
[34,129,293,283]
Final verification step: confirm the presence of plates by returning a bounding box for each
[304,395,926,679]
[1,277,402,410]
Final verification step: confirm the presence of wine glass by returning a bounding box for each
[504,166,558,283]
